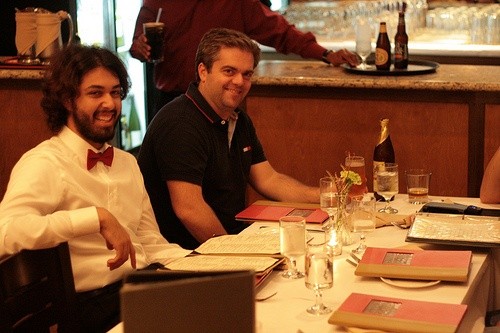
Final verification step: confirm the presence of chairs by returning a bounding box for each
[0,242,90,333]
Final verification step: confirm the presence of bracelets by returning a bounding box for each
[321,49,334,64]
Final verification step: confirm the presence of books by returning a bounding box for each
[156,232,313,289]
[235,200,329,224]
[404,201,500,247]
[354,245,472,283]
[328,292,468,333]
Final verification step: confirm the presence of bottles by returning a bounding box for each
[375,21,391,70]
[372,118,396,202]
[394,12,408,69]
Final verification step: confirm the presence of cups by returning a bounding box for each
[409,0,500,45]
[405,169,432,204]
[143,22,165,64]
[283,0,400,40]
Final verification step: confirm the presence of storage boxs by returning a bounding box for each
[118,260,259,333]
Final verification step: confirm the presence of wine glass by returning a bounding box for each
[278,216,307,281]
[319,177,340,231]
[344,156,369,214]
[373,162,399,214]
[303,243,334,316]
[351,195,376,254]
[353,23,372,69]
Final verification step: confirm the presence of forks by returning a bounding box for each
[391,221,412,230]
[255,291,278,302]
[305,237,314,244]
[350,253,361,263]
[346,259,357,267]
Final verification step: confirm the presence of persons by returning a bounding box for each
[0,46,195,333]
[129,0,363,132]
[480,146,500,203]
[137,28,368,252]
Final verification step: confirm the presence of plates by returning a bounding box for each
[380,277,442,289]
[343,326,386,333]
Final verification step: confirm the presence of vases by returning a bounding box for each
[330,194,360,244]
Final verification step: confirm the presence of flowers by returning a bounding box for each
[325,150,363,197]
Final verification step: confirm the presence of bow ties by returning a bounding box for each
[87,145,114,170]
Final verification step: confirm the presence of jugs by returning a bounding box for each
[14,6,35,65]
[34,8,74,66]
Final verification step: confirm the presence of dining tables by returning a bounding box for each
[107,187,499,333]
